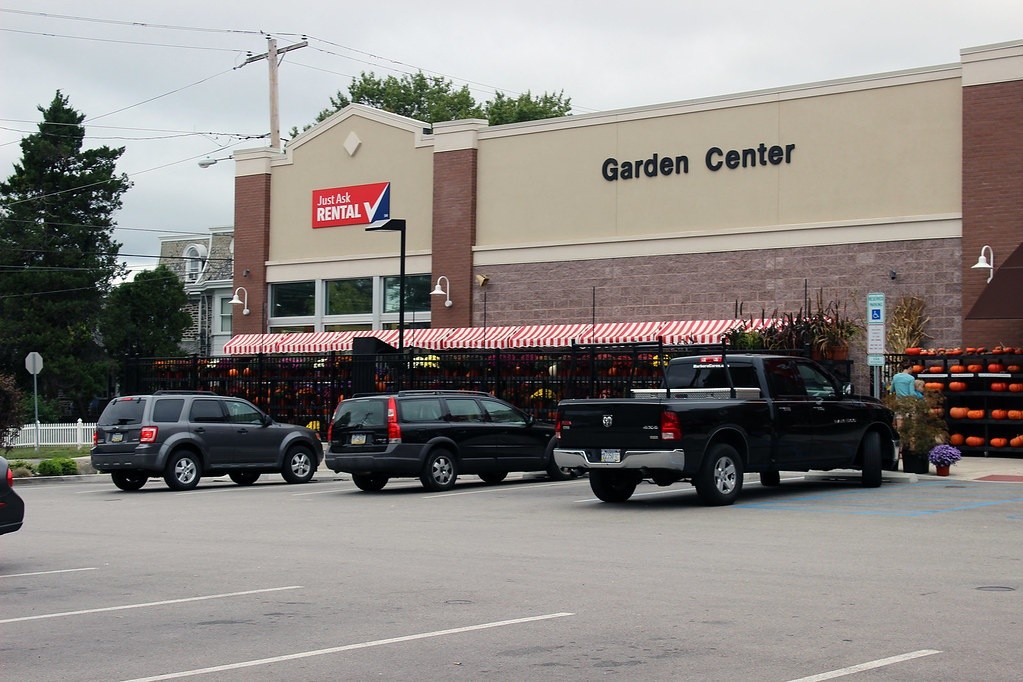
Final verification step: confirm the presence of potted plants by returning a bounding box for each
[883,389,948,474]
[724,299,866,360]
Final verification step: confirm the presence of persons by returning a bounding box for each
[888,362,927,454]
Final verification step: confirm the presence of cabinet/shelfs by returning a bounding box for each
[905,350,1023,457]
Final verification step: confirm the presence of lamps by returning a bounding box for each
[429,275,452,307]
[228,287,249,315]
[971,244,993,284]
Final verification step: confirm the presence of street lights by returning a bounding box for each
[364,218,407,390]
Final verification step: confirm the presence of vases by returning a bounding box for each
[937,465,949,476]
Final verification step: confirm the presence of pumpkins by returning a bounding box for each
[608,366,618,377]
[228,366,389,417]
[904,344,1023,448]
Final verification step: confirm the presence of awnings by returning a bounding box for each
[224,316,832,355]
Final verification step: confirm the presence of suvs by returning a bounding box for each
[324,390,585,492]
[90,390,325,492]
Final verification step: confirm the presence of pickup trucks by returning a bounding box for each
[553,336,903,508]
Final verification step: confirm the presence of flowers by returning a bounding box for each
[927,444,961,467]
